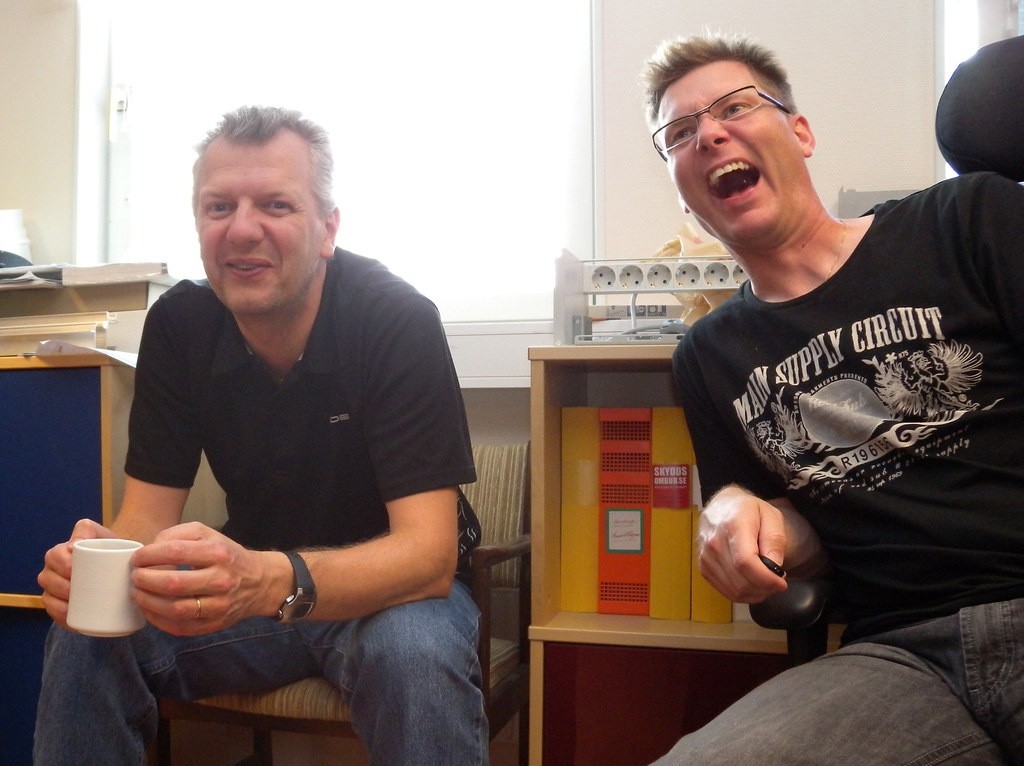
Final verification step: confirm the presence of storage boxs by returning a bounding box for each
[559,401,738,624]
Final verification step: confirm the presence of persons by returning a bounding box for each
[645,36,1024,765]
[33,105,489,766]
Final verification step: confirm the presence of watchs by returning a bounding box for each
[271,550,317,625]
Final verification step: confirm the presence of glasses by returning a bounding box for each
[652,85,791,163]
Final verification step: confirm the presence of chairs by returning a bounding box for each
[154,442,530,766]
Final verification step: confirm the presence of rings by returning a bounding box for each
[192,596,201,619]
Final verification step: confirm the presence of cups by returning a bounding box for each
[66,539,146,638]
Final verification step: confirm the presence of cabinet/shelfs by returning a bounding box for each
[527,347,848,766]
[0,353,134,766]
